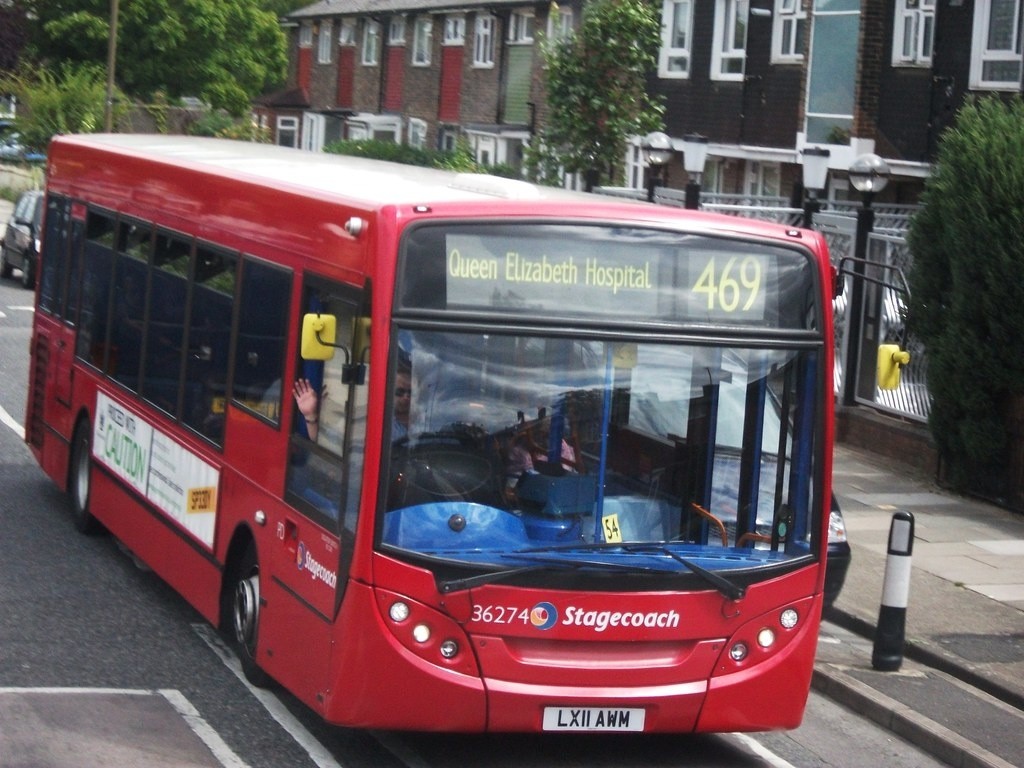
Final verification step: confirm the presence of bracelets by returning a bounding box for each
[305,418,318,424]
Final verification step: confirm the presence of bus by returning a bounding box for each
[25,132,913,733]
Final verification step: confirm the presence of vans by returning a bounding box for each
[527,338,851,622]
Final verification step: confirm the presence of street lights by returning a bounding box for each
[642,132,676,204]
[526,102,535,135]
[843,152,892,406]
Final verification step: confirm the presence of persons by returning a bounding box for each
[292,358,499,508]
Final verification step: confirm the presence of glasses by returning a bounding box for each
[395,386,421,397]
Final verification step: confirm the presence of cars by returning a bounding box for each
[0,190,45,290]
[0,121,47,171]
